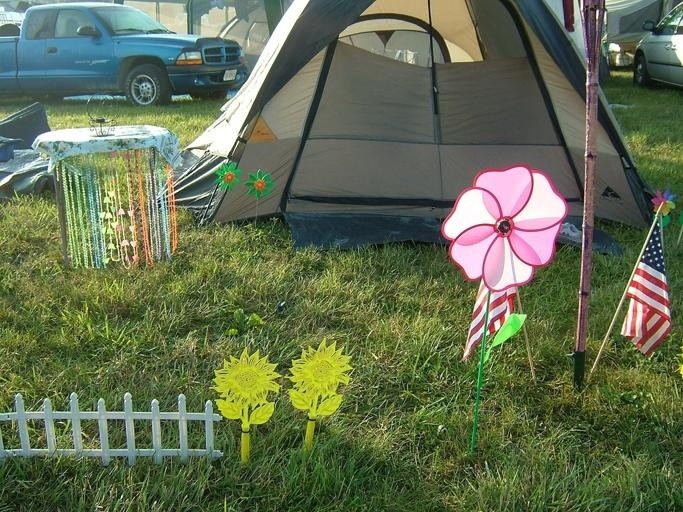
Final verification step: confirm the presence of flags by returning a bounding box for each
[613,212,674,359]
[458,275,518,365]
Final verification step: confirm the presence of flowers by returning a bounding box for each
[203,158,237,227]
[290,340,354,451]
[214,346,281,464]
[245,170,274,227]
[444,160,567,453]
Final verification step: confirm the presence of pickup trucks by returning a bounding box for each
[0,2,249,106]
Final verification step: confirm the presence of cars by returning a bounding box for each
[630,0,683,93]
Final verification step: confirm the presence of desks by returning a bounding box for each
[38,125,177,275]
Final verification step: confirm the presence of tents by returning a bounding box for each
[144,0,663,260]
[600,0,683,72]
